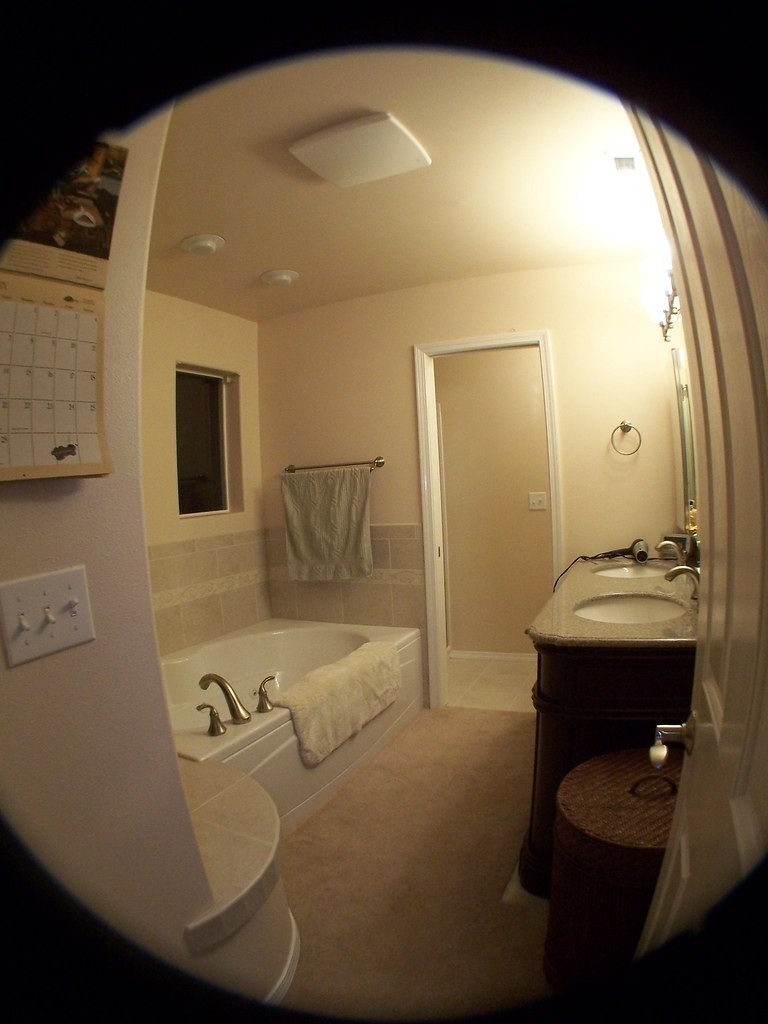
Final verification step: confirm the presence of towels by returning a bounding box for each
[268,639,402,768]
[279,465,375,584]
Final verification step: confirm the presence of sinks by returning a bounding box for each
[592,565,675,579]
[573,593,689,624]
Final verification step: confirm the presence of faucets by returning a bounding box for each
[654,540,687,567]
[196,672,253,726]
[663,565,703,599]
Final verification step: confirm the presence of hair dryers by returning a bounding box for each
[590,538,650,565]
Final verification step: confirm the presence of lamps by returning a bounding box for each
[284,108,434,193]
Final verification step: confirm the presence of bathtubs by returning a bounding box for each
[158,616,426,847]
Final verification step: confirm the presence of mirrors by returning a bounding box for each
[671,337,700,537]
